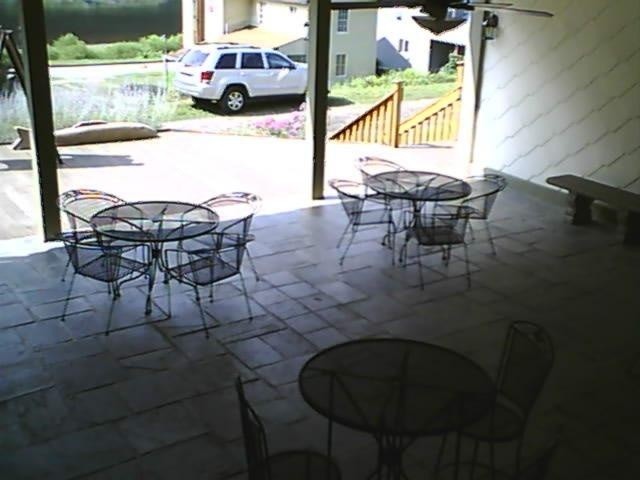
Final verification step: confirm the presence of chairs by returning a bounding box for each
[231,372,344,480]
[437,322,556,478]
[330,153,507,292]
[51,187,261,340]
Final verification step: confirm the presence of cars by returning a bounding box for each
[172,42,307,114]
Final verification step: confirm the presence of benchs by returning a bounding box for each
[546,174,640,243]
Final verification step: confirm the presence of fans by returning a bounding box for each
[328,0,555,35]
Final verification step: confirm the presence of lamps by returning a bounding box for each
[412,2,466,36]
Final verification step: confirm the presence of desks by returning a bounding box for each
[298,336,497,479]
